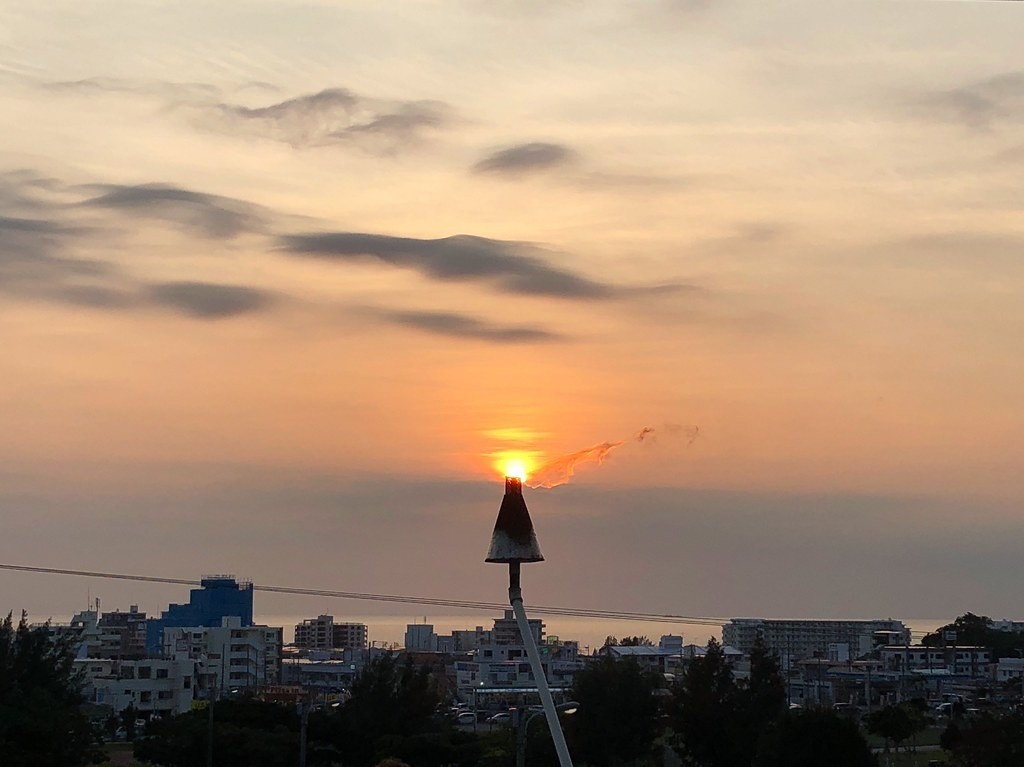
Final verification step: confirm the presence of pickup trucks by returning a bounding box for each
[926,693,959,709]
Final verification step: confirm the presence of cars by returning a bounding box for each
[486,712,510,723]
[935,703,952,716]
[832,703,863,713]
[459,713,475,724]
[116,725,140,737]
[136,719,145,727]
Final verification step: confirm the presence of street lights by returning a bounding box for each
[516,701,580,767]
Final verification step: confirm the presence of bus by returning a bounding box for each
[473,688,574,713]
